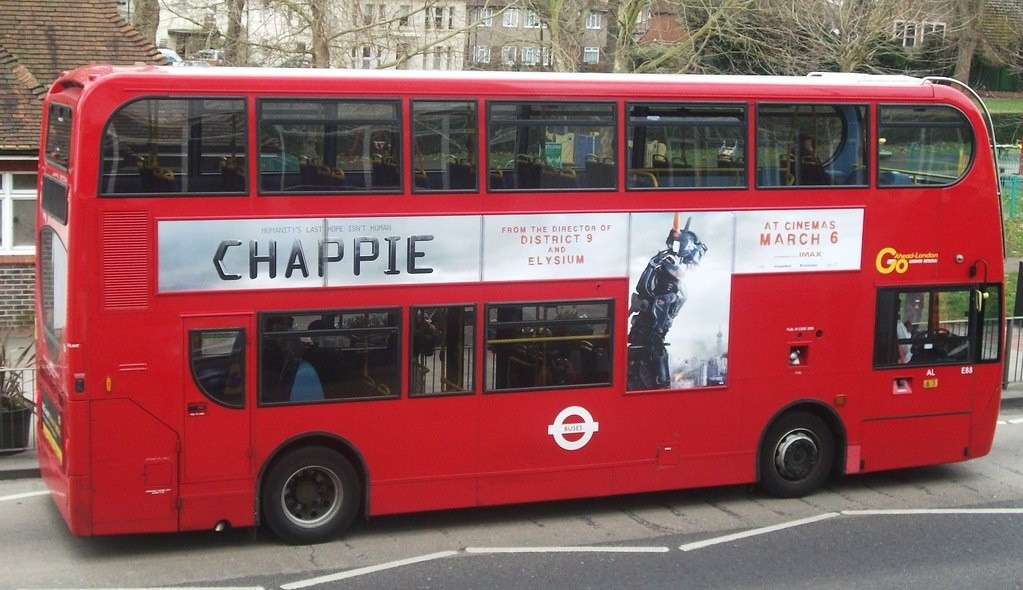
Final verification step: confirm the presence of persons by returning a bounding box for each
[264,315,364,399]
[790,134,839,185]
[897,298,915,365]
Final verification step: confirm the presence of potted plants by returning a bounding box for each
[0,334,37,456]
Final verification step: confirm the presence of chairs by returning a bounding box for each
[364,376,390,396]
[601,158,616,188]
[220,156,245,192]
[489,166,506,189]
[779,154,800,186]
[372,154,400,186]
[516,154,580,189]
[414,167,429,190]
[513,341,609,385]
[671,157,691,184]
[443,154,476,190]
[584,154,601,185]
[798,156,828,185]
[297,154,351,191]
[631,172,659,188]
[135,155,179,194]
[651,154,669,180]
[716,153,746,177]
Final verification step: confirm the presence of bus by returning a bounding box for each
[34,62,1008,546]
